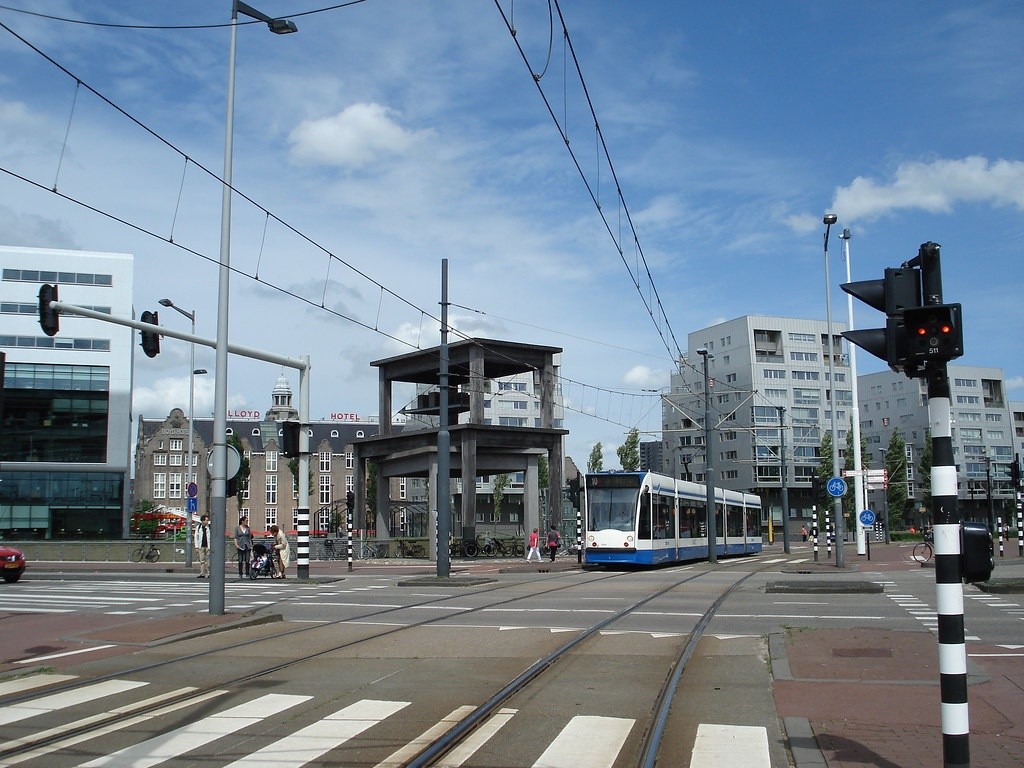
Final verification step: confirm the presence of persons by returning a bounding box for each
[802,524,814,542]
[234,516,290,580]
[526,528,544,564]
[194,515,211,579]
[614,509,630,523]
[546,525,560,563]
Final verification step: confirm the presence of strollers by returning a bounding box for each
[249,544,277,579]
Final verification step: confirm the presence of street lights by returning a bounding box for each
[823,214,845,568]
[159,298,208,569]
[878,448,890,544]
[983,457,994,556]
[696,348,717,563]
[969,479,976,523]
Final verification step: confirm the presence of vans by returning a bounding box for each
[829,522,848,541]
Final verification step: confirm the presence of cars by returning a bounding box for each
[0,545,26,583]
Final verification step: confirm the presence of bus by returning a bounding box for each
[575,468,761,568]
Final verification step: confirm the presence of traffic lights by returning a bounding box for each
[903,303,964,363]
[565,479,575,503]
[345,492,354,510]
[1005,462,1018,487]
[839,267,922,373]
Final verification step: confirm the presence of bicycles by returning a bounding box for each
[913,534,935,563]
[393,540,426,558]
[132,542,160,564]
[359,542,386,560]
[449,536,524,558]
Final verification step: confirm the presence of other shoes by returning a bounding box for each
[273,575,282,579]
[527,560,531,564]
[550,558,555,563]
[539,560,543,563]
[282,575,286,578]
[197,574,205,578]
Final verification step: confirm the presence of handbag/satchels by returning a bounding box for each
[526,545,531,551]
